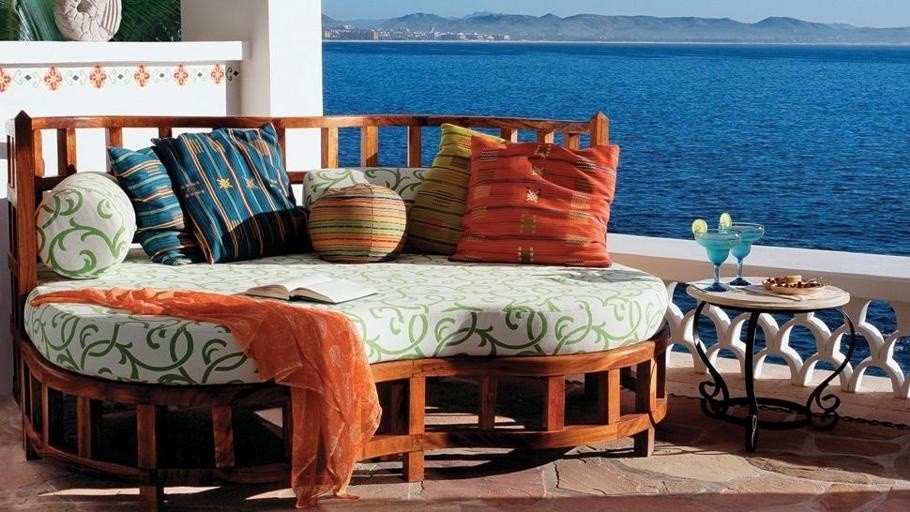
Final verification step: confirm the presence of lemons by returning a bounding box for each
[691,219,708,237]
[720,212,733,228]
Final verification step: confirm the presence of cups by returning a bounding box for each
[787,275,801,284]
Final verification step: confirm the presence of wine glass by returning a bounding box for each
[694,223,765,292]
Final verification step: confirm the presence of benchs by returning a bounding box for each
[5,109,672,512]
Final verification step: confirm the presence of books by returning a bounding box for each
[246,274,377,304]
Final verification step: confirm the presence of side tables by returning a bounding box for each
[685,279,857,456]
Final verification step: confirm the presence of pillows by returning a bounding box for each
[448,136,621,267]
[303,166,433,211]
[306,183,408,263]
[37,171,135,279]
[106,144,202,268]
[152,122,310,265]
[408,124,508,256]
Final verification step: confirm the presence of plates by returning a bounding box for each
[769,283,826,295]
